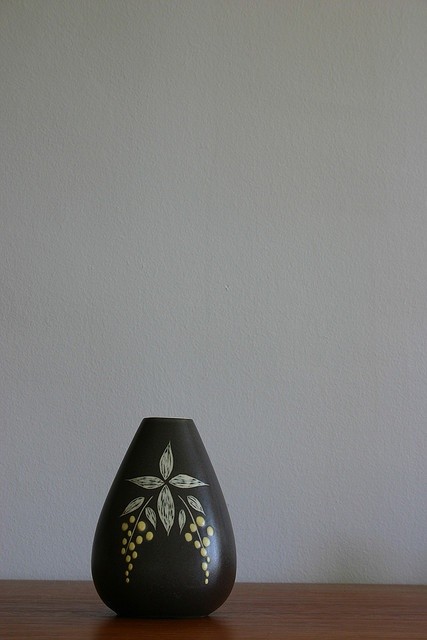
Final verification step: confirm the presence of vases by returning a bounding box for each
[90,417,236,618]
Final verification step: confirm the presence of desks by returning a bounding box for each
[0,578,427,639]
